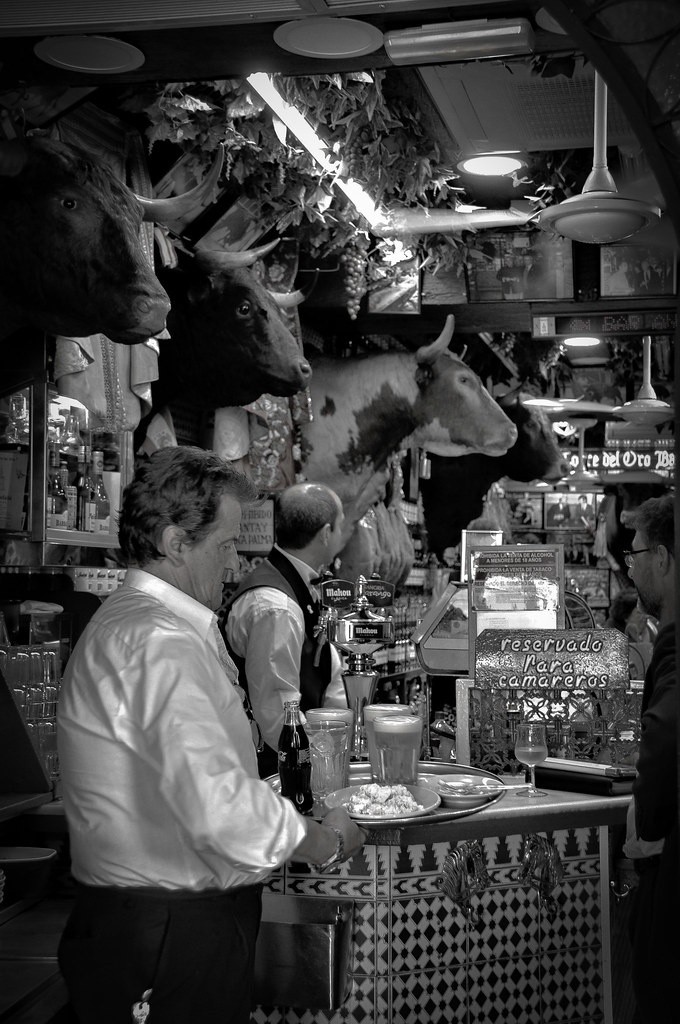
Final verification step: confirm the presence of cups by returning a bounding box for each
[306,707,353,772]
[6,395,29,443]
[372,715,423,784]
[0,646,63,779]
[303,721,348,801]
[362,704,412,779]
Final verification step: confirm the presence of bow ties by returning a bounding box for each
[309,573,330,585]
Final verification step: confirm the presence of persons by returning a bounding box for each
[222,481,349,776]
[619,493,680,1024]
[548,495,593,526]
[56,447,367,1023]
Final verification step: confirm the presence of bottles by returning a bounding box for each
[276,701,314,813]
[46,405,110,534]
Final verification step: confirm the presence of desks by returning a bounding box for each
[262,784,636,1024]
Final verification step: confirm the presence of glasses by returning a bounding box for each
[623,549,649,568]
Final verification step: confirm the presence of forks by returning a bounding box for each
[438,779,533,795]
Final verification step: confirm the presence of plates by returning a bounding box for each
[324,784,441,819]
[420,774,503,803]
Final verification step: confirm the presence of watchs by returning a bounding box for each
[308,827,344,875]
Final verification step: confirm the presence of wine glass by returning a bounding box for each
[514,723,549,798]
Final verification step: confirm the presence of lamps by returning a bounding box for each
[382,16,537,65]
[615,335,676,426]
[561,419,601,486]
[538,69,661,245]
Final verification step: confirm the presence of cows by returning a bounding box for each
[0,137,321,407]
[295,313,570,573]
